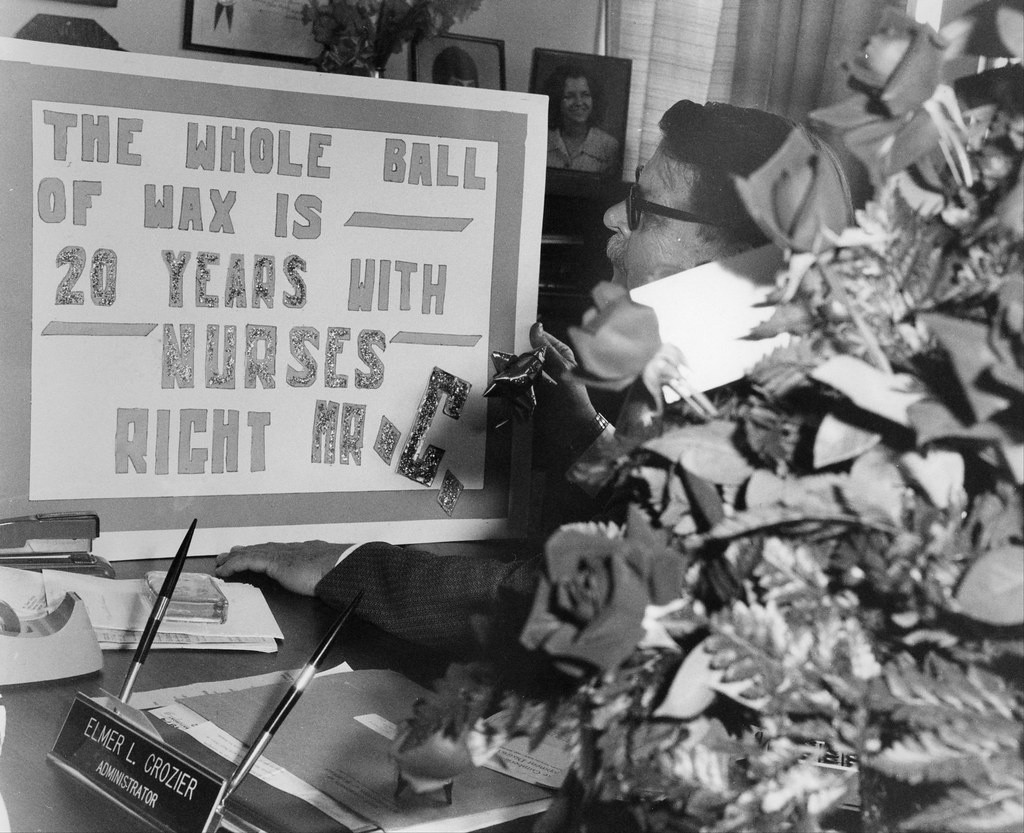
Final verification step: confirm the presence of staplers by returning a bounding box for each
[0,511,117,579]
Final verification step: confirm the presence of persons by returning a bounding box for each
[433,46,479,88]
[547,66,620,173]
[216,99,796,646]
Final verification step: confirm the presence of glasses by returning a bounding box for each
[627,165,746,233]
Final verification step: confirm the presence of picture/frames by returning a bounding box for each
[531,47,632,182]
[413,30,505,90]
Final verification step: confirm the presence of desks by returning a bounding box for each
[0,554,449,833]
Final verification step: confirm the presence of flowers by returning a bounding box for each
[382,1,1024,833]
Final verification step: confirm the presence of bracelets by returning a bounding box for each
[570,413,609,454]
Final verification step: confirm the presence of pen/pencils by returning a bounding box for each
[114,517,200,714]
[215,587,369,813]
